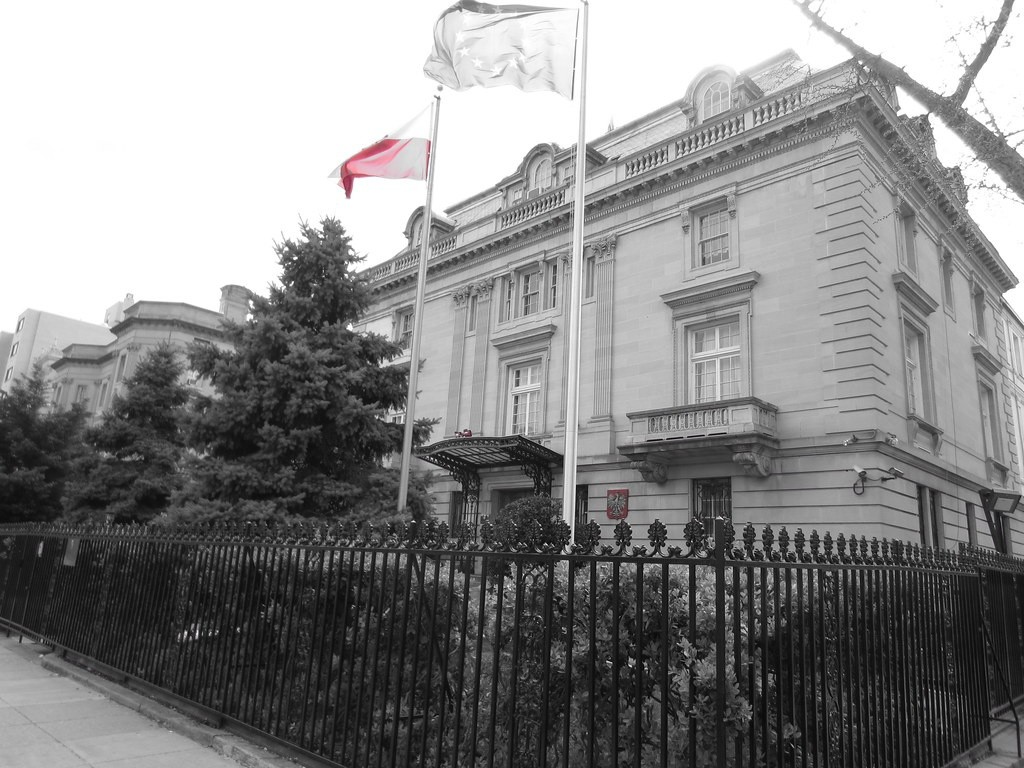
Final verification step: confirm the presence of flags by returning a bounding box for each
[328,101,434,200]
[424,0,580,100]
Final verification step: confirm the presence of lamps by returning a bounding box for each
[841,435,858,446]
[885,433,898,445]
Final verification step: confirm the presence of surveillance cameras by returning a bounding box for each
[852,465,867,476]
[888,467,903,477]
